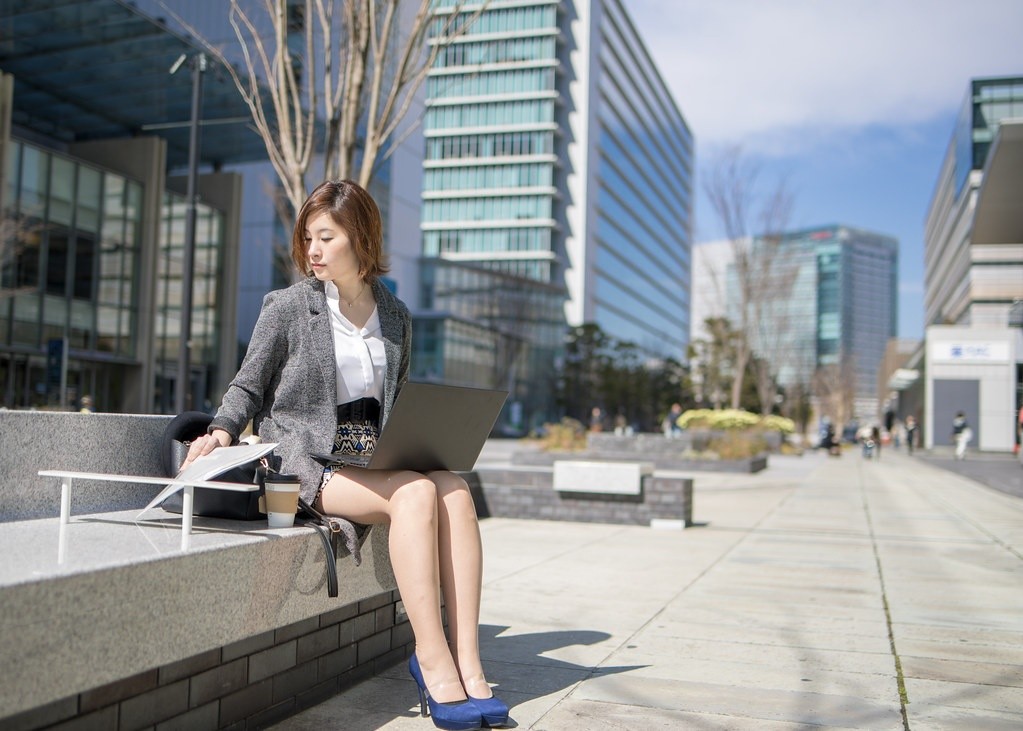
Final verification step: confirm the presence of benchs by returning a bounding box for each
[0,410,450,731]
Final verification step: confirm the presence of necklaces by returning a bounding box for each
[340,283,366,308]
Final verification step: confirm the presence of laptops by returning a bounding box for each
[309,381,511,473]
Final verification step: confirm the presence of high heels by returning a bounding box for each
[465,689,509,726]
[408,652,482,731]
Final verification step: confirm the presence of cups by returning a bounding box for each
[264,474,301,529]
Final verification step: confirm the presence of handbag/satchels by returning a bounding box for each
[160,438,282,521]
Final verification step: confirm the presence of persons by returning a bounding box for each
[80,395,95,412]
[661,403,684,439]
[179,179,510,731]
[614,414,633,435]
[821,411,972,460]
